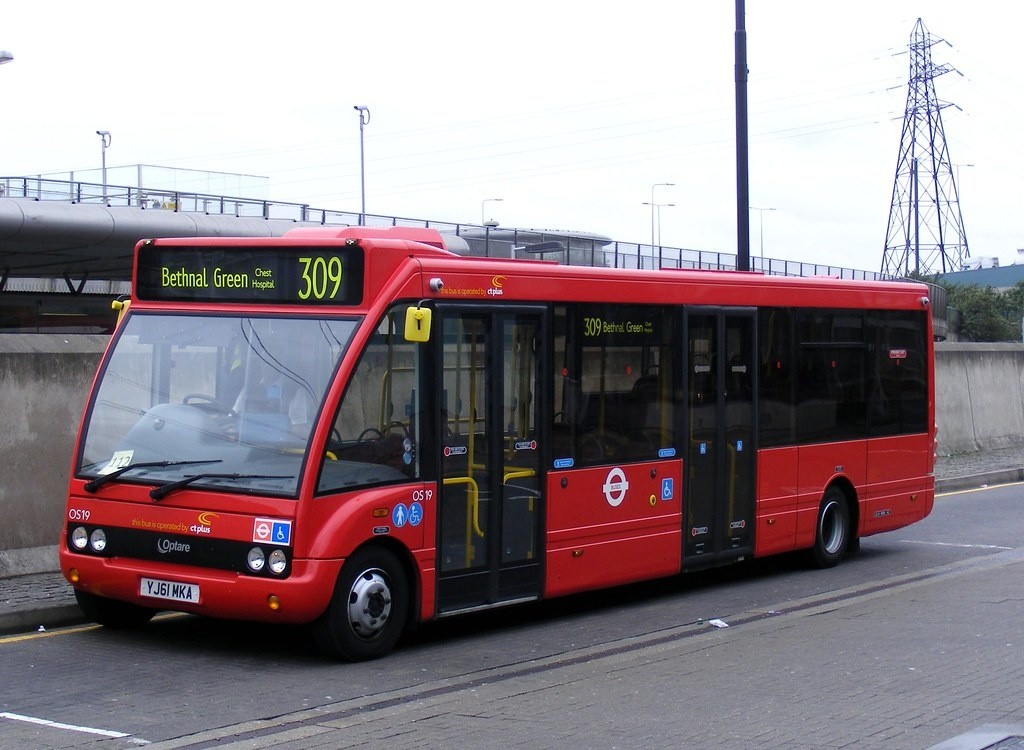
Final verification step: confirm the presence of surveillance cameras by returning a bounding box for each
[96,130,109,135]
[354,105,368,110]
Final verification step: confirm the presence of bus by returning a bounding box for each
[58,226,940,662]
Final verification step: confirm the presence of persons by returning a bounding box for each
[221,344,310,453]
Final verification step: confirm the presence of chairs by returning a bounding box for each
[552,354,881,464]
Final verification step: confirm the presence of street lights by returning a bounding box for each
[643,202,676,268]
[481,198,502,228]
[749,206,777,270]
[651,183,676,269]
